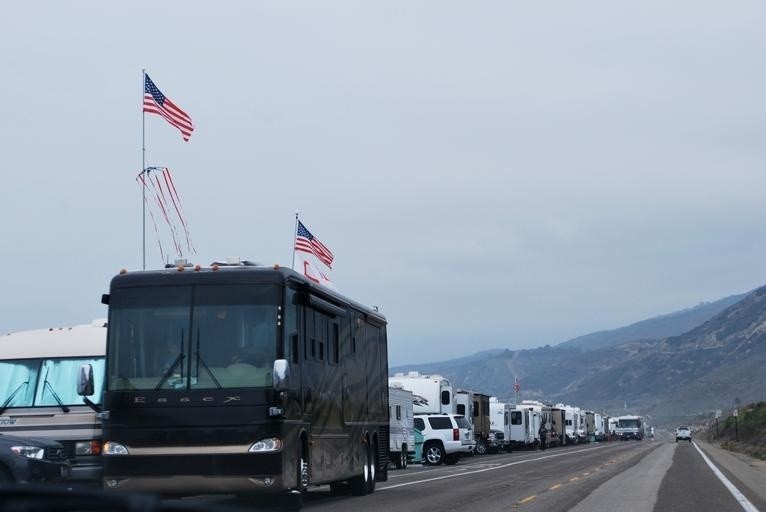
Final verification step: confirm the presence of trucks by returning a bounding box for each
[388,370,656,469]
[675,425,693,443]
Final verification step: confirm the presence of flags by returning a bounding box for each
[142,72,196,144]
[294,220,338,272]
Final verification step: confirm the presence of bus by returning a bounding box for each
[76,260,391,512]
[0,317,108,495]
